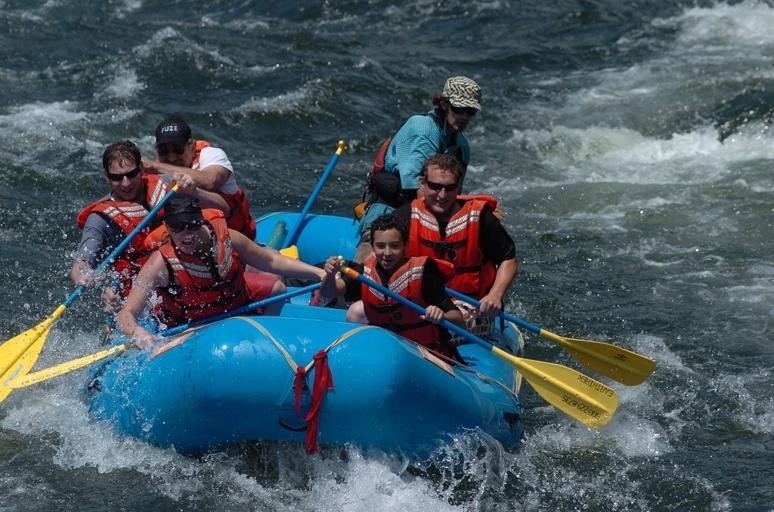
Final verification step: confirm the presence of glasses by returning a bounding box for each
[156,146,186,155]
[107,167,140,181]
[170,224,201,231]
[425,177,458,191]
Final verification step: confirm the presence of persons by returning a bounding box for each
[358,77,482,237]
[117,192,339,355]
[71,140,231,311]
[137,117,256,244]
[319,213,462,367]
[392,154,518,348]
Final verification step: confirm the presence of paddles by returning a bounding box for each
[0,175,190,406]
[333,255,621,429]
[443,288,656,387]
[7,282,321,389]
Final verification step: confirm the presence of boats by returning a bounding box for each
[80,212,524,469]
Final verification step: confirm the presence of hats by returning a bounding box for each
[443,77,482,111]
[164,193,202,225]
[155,118,193,142]
